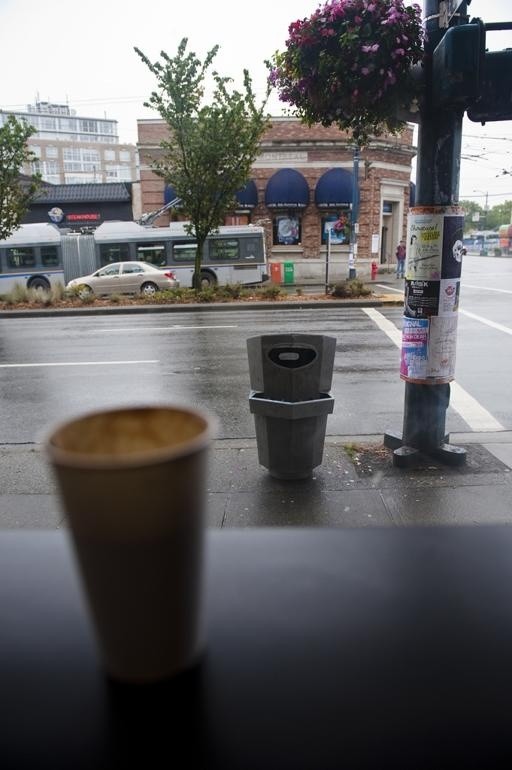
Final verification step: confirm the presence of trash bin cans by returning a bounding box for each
[271,261,295,284]
[247,333,337,480]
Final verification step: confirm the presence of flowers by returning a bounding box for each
[263,0,429,148]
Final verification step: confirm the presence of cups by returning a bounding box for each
[43,406,222,686]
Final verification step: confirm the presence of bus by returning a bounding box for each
[0,221,269,289]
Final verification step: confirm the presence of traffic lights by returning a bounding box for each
[364,159,372,179]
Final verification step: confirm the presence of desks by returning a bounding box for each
[0,525,512,770]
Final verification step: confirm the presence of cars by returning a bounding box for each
[462,235,502,257]
[66,261,179,301]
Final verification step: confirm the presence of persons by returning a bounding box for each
[409,234,422,272]
[395,240,407,279]
[334,214,347,240]
